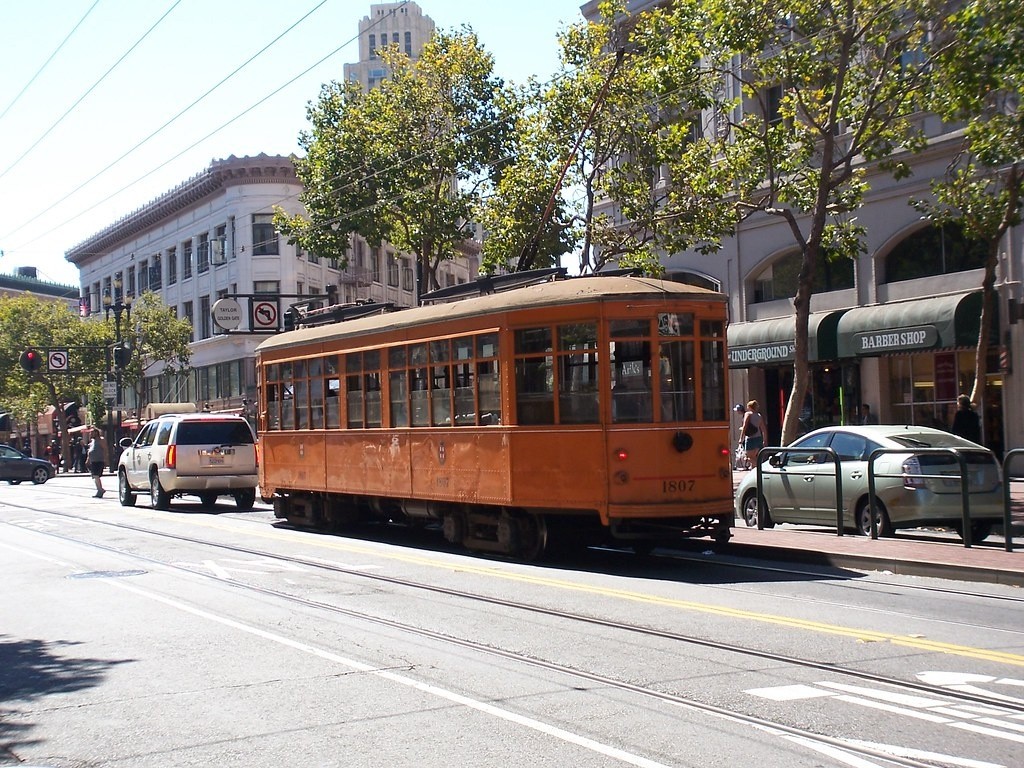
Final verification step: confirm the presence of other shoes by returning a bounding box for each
[74,471,79,473]
[81,471,89,473]
[92,489,106,498]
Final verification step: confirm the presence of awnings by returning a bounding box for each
[836,288,1000,364]
[725,304,861,369]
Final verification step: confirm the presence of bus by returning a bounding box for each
[254,256,735,562]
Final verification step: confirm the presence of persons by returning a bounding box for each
[73,436,90,473]
[860,404,876,425]
[84,429,108,498]
[950,394,983,446]
[732,400,767,471]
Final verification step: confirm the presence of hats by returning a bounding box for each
[733,404,744,411]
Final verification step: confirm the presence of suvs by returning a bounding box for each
[117,413,260,511]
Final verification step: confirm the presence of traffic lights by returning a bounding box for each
[20,349,43,371]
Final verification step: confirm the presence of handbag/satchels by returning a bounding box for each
[735,444,751,468]
[745,423,758,436]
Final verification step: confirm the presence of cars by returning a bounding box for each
[0,444,54,485]
[736,424,1007,541]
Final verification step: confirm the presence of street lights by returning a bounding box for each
[103,273,132,472]
